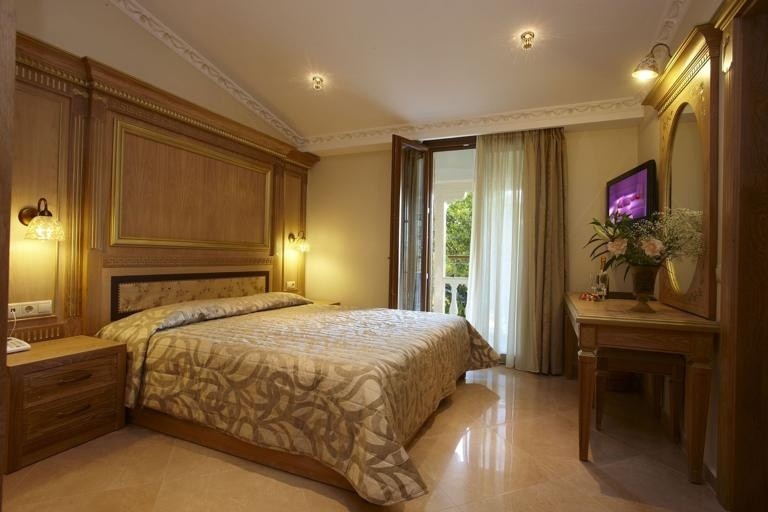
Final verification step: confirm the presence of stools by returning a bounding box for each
[592,357,663,427]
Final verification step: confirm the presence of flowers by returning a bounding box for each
[582,208,678,281]
[668,205,704,258]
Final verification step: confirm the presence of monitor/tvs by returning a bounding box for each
[606,160,659,225]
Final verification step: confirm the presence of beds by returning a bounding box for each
[93,291,500,492]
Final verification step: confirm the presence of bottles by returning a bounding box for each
[597,256,609,298]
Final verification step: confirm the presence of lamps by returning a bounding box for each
[288,230,307,251]
[18,197,65,241]
[632,42,672,82]
[313,76,323,90]
[521,31,534,49]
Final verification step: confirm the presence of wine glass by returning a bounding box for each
[589,271,608,302]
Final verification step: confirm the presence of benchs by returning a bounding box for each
[564,291,719,484]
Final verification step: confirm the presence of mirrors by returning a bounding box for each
[641,23,719,320]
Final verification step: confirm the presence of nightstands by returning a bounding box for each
[7,334,127,473]
[311,298,340,305]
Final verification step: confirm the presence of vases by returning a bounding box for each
[626,260,660,314]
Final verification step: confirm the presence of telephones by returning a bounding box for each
[7,337,31,354]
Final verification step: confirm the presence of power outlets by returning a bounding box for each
[287,281,295,290]
